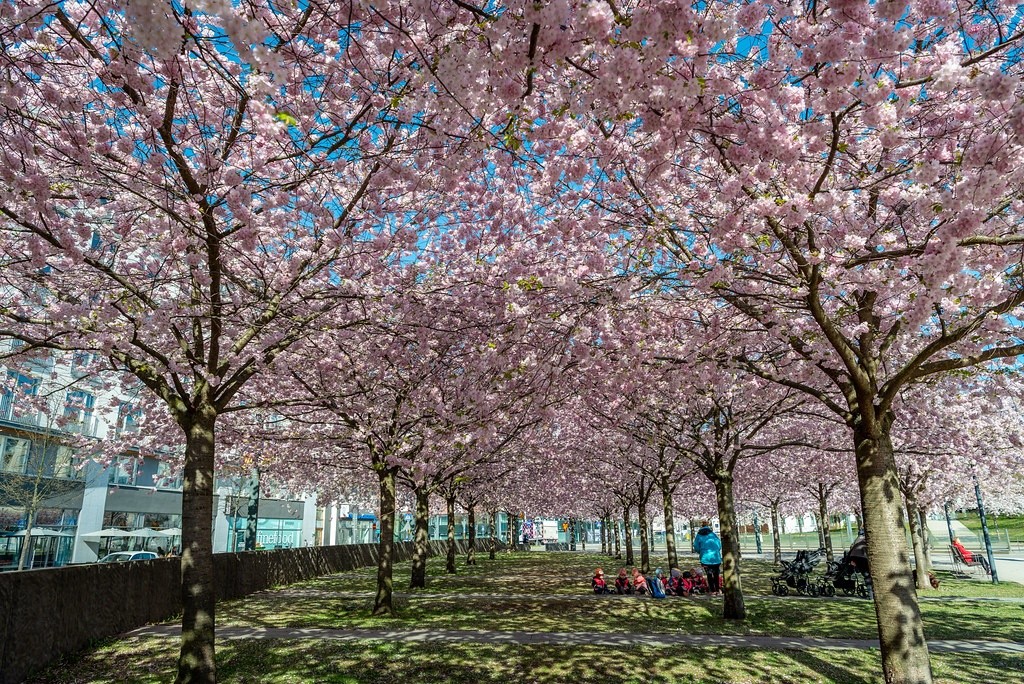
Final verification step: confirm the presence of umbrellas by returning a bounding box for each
[6,526,182,568]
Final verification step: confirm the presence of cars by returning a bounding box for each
[102,549,160,562]
[653,532,661,543]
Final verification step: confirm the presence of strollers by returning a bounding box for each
[771,546,868,597]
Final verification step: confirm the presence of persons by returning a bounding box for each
[693,521,722,592]
[953,537,992,575]
[157,547,165,556]
[849,533,873,600]
[172,547,177,555]
[591,567,724,596]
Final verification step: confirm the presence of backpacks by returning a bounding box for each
[646,575,666,598]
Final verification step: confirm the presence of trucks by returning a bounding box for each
[519,520,557,545]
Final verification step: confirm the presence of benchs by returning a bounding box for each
[949,544,990,580]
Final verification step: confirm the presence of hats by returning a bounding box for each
[632,568,638,576]
[596,568,604,576]
[671,568,681,577]
[682,571,692,578]
[619,568,626,576]
[656,568,663,575]
